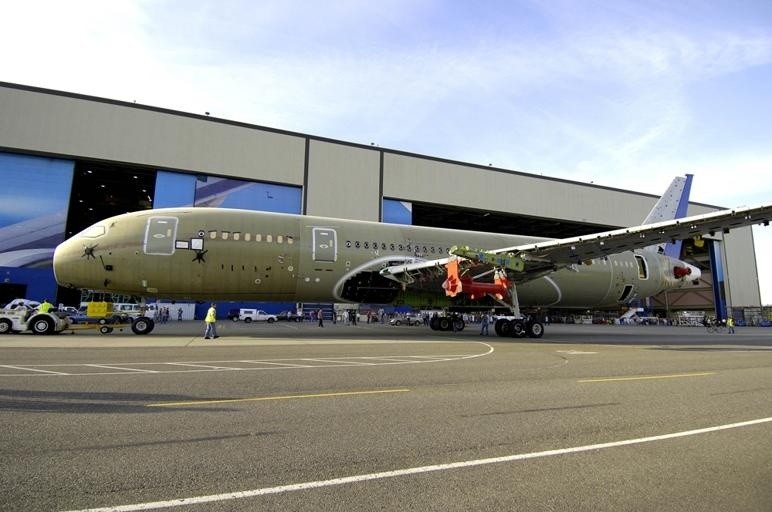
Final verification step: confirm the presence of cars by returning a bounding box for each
[732,319,772,327]
[680,320,691,327]
[390,313,425,326]
[0,303,158,334]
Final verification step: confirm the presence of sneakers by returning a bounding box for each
[204,335,221,339]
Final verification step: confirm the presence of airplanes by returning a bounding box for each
[48,172,772,341]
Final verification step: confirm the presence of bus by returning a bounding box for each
[678,316,705,327]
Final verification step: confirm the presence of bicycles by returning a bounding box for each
[707,322,723,334]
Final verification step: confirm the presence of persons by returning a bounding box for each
[16,301,29,311]
[479,311,489,336]
[284,305,497,328]
[28,299,57,315]
[540,311,767,334]
[204,302,220,339]
[148,303,185,326]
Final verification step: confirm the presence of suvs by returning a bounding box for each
[225,307,305,324]
